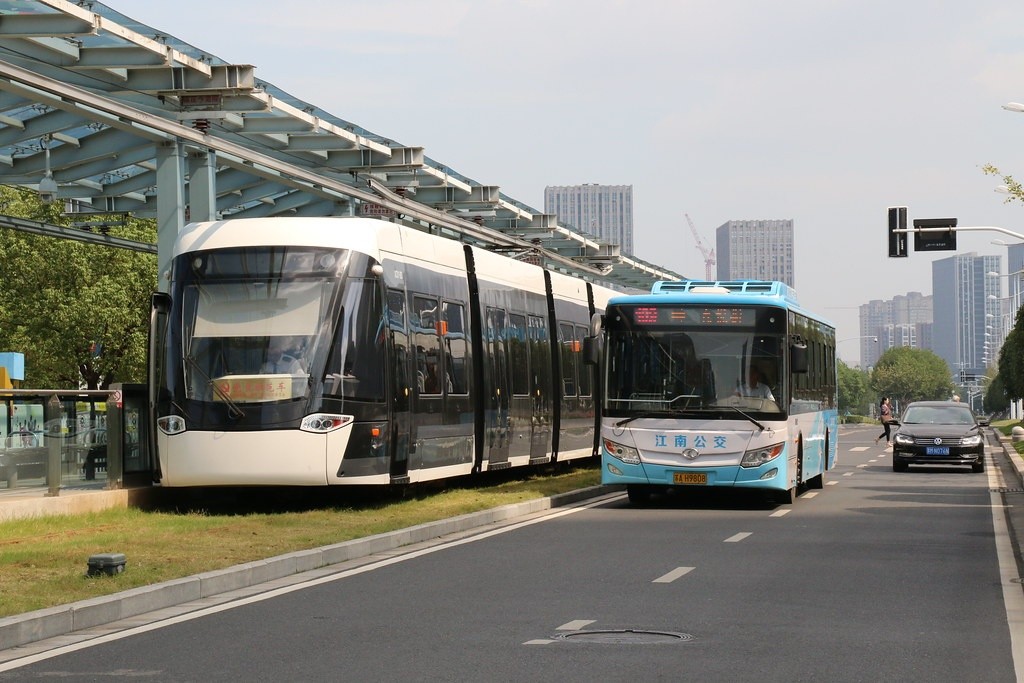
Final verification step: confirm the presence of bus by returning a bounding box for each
[146,216,625,487]
[600,278,839,505]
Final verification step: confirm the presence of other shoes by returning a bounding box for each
[886,442,893,448]
[873,438,880,448]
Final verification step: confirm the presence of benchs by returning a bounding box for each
[4,428,132,478]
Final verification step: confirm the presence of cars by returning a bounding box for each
[893,401,990,472]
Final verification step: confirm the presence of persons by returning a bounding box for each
[260,340,303,374]
[875,397,893,447]
[732,365,775,401]
